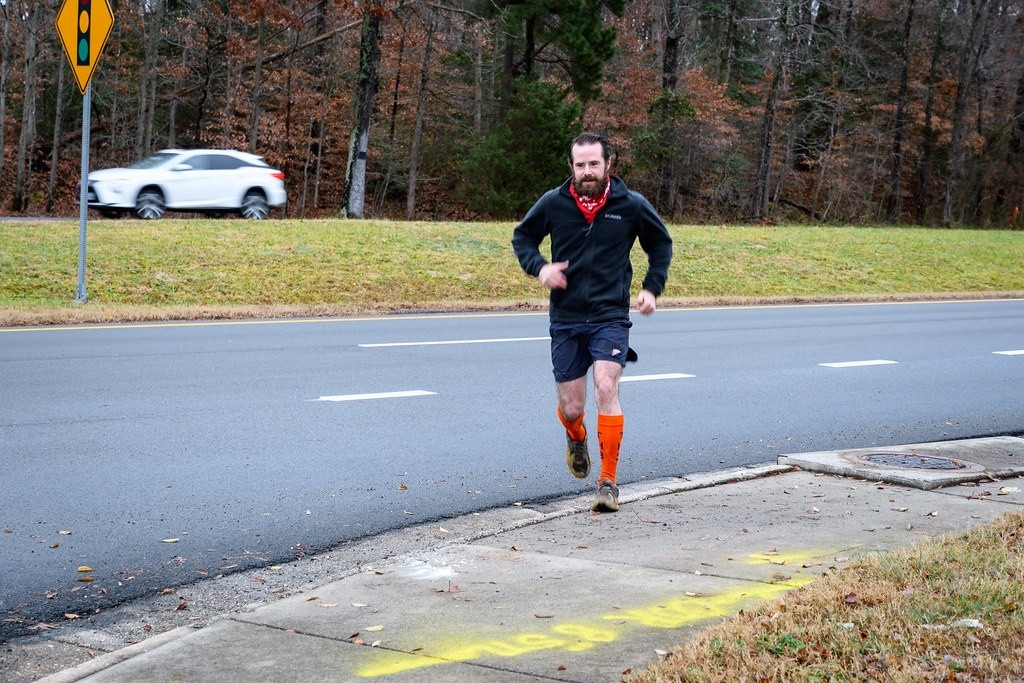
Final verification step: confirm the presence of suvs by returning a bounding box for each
[75,148,287,220]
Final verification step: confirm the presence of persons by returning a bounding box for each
[512,133,673,511]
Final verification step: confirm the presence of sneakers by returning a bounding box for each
[591,478,620,512]
[566,421,591,479]
